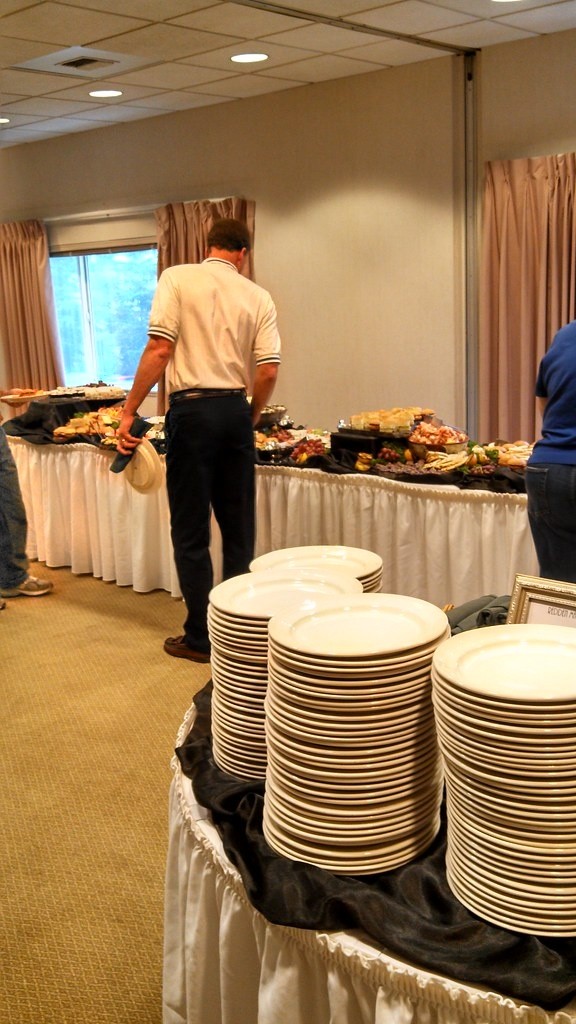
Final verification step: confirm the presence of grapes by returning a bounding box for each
[471,465,495,474]
[291,440,323,459]
[378,448,400,461]
[270,431,293,441]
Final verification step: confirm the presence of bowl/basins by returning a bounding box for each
[255,404,287,426]
[407,435,469,458]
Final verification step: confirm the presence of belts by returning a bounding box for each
[169,389,246,406]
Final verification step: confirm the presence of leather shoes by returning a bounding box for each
[164,635,211,663]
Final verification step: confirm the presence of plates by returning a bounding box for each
[206,571,362,783]
[124,437,162,495]
[261,593,451,877]
[248,544,384,594]
[0,394,49,402]
[429,623,576,938]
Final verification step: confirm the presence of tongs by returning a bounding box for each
[431,416,467,434]
[89,420,106,441]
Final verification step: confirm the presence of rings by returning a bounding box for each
[121,439,126,442]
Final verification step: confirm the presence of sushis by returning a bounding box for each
[50,389,85,398]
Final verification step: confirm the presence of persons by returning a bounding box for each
[0,415,53,610]
[116,219,282,663]
[524,320,576,584]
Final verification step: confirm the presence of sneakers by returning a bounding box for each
[0,575,52,598]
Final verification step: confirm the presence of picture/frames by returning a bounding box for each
[504,571,575,628]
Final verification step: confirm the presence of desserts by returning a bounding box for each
[350,408,435,433]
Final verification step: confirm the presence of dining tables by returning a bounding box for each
[3,429,542,613]
[159,677,576,1024]
